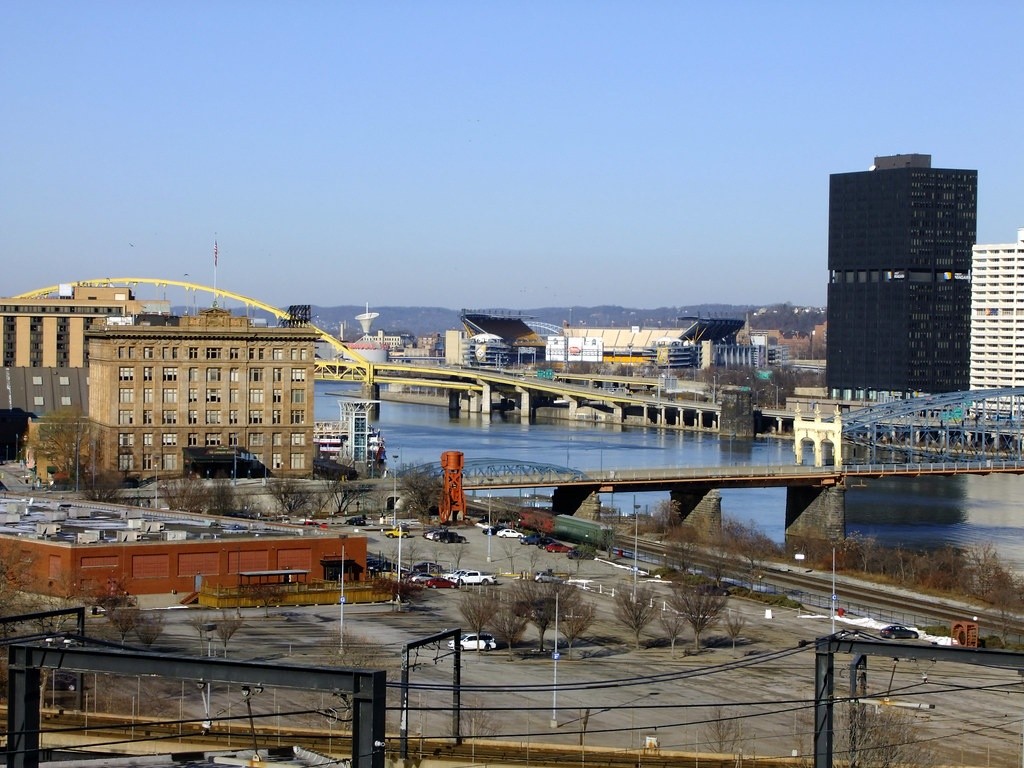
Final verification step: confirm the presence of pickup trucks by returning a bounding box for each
[454,570,494,586]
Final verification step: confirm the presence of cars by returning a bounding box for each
[426,578,458,589]
[497,529,524,538]
[44,672,77,691]
[519,534,541,545]
[537,538,556,549]
[367,556,442,578]
[880,625,919,639]
[482,525,507,535]
[441,571,456,580]
[386,526,410,539]
[546,542,572,553]
[694,585,732,597]
[448,633,497,652]
[346,516,365,526]
[423,529,467,543]
[566,550,595,560]
[412,573,434,583]
[535,571,560,583]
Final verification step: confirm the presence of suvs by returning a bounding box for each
[305,521,319,529]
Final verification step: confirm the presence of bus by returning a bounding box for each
[260,517,291,525]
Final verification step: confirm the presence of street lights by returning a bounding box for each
[551,581,558,729]
[154,464,158,509]
[338,534,349,656]
[634,505,641,603]
[830,535,838,635]
[487,478,493,562]
[202,624,218,719]
[393,455,398,529]
[234,435,239,486]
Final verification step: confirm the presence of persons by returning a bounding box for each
[491,572,497,587]
